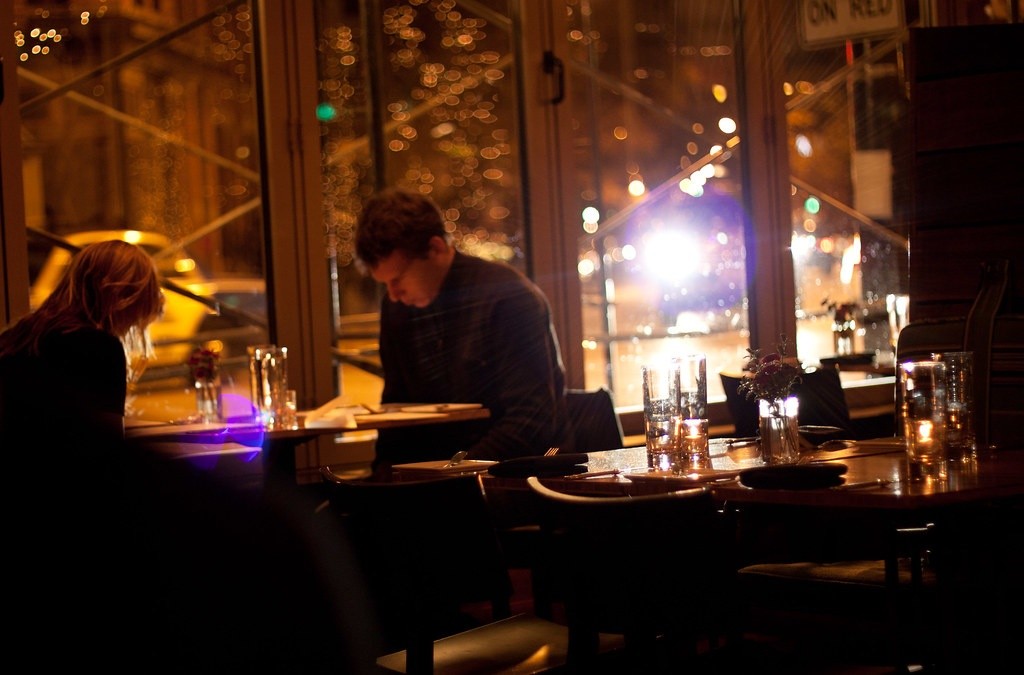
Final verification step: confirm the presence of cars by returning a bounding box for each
[629,191,750,330]
[27,228,267,382]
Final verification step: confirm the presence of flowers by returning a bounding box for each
[824,296,861,326]
[742,344,796,401]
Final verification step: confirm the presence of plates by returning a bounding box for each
[740,461,846,484]
[623,467,741,483]
[391,459,499,473]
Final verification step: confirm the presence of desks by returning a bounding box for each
[499,438,1006,674]
[123,404,492,485]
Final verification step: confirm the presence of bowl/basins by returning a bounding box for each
[796,424,843,447]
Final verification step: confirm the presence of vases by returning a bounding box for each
[833,326,855,358]
[756,391,802,461]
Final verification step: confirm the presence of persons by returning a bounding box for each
[352,192,567,460]
[0,240,165,437]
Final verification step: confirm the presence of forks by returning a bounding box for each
[543,447,559,456]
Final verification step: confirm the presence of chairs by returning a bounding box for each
[0,390,977,675]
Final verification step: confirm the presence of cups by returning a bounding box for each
[640,358,709,461]
[897,360,951,481]
[247,344,298,430]
[931,351,977,461]
